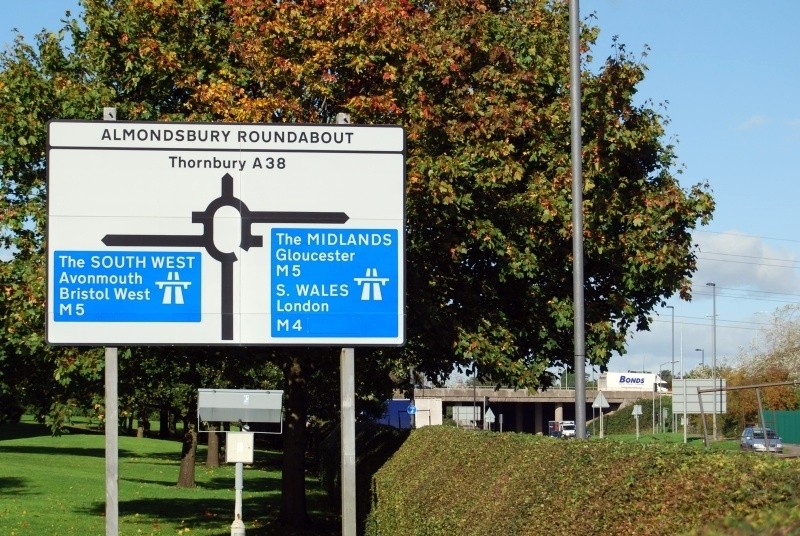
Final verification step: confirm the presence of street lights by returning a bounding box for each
[660,361,679,432]
[696,349,704,378]
[666,306,674,379]
[706,282,717,439]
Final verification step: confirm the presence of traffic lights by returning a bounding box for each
[485,397,489,406]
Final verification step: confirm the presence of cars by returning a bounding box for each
[740,426,783,454]
[586,430,591,439]
[550,431,564,438]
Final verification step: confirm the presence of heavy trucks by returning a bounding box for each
[597,371,668,391]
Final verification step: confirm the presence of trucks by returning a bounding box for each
[549,421,576,437]
